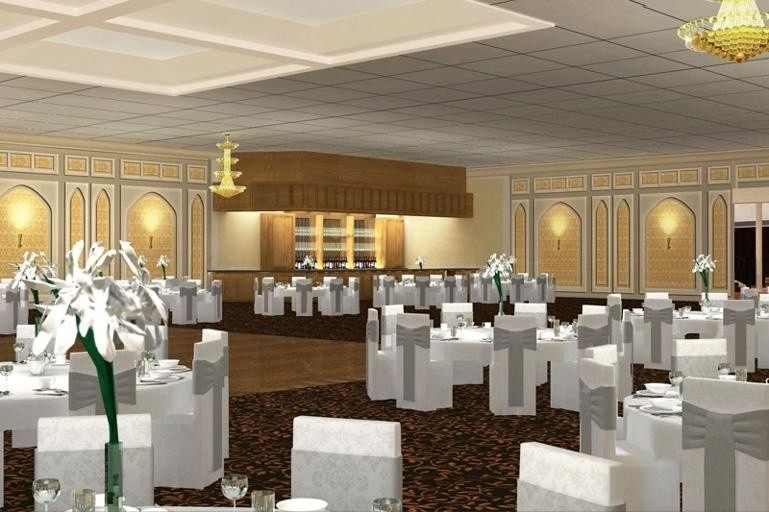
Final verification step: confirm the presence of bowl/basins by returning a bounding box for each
[276,497,328,512]
[94,492,125,512]
[719,374,737,381]
[140,357,186,386]
[648,398,680,410]
[644,382,672,395]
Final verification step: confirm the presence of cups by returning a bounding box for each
[71,488,96,512]
[274,281,291,290]
[39,378,50,390]
[251,489,276,512]
[51,351,66,363]
[372,496,403,512]
[735,368,747,382]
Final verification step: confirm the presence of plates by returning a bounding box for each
[635,390,680,397]
[32,387,64,396]
[137,505,169,512]
[630,308,644,316]
[639,405,683,415]
[431,315,494,343]
[51,362,69,365]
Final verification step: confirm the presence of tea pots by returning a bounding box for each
[25,351,50,377]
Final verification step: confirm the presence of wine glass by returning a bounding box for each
[396,277,415,286]
[220,473,249,511]
[1,362,14,394]
[13,343,25,363]
[698,299,721,321]
[537,315,578,343]
[31,477,63,512]
[676,305,691,319]
[668,368,684,398]
[760,303,769,319]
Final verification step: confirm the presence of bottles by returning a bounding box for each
[294,255,375,270]
